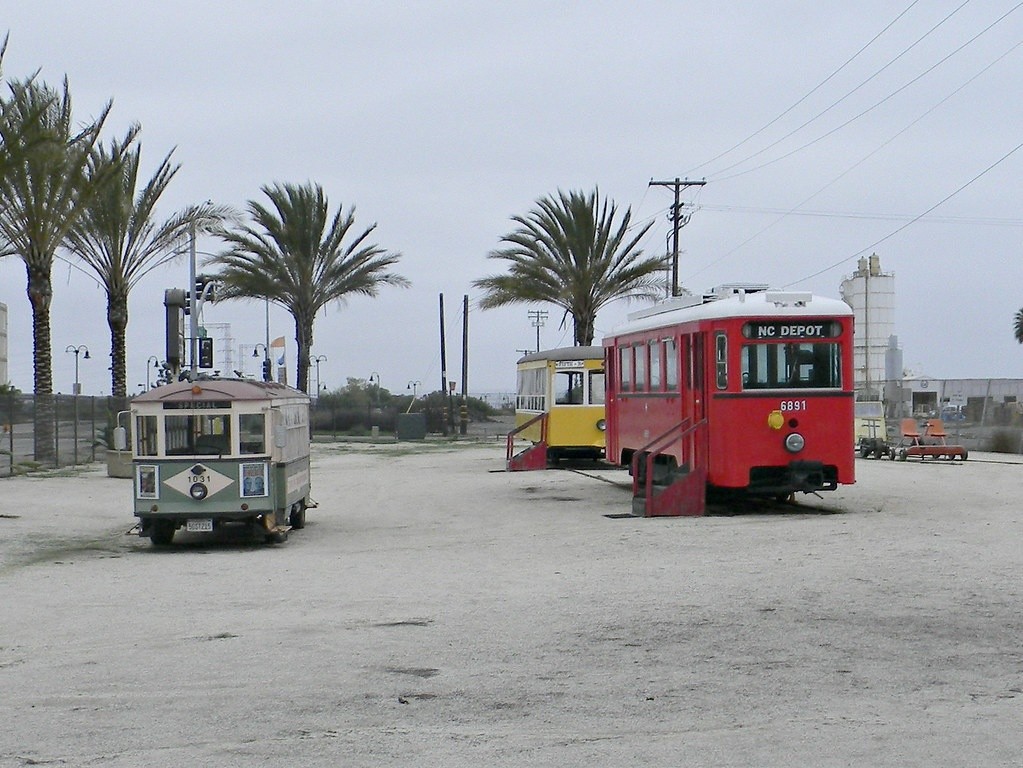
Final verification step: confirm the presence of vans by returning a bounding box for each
[943,404,964,419]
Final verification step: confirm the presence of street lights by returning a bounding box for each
[309,354,327,397]
[852,251,882,398]
[369,371,380,388]
[64,344,90,465]
[137,355,160,392]
[253,343,272,382]
[406,380,422,396]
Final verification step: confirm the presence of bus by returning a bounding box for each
[126,377,319,546]
[514,344,605,463]
[601,281,856,503]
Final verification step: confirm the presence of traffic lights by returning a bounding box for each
[262,361,270,378]
[184,290,192,314]
